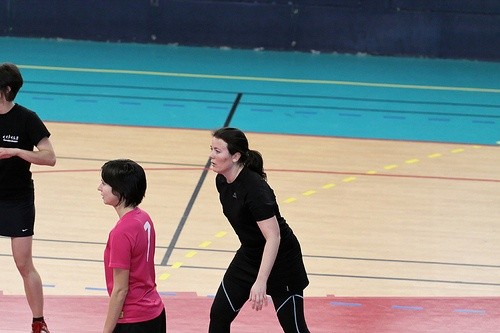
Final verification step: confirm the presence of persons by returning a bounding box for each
[208,128,311,333]
[97,159,166,333]
[0,62,56,333]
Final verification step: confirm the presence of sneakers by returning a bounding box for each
[32,320,48,332]
